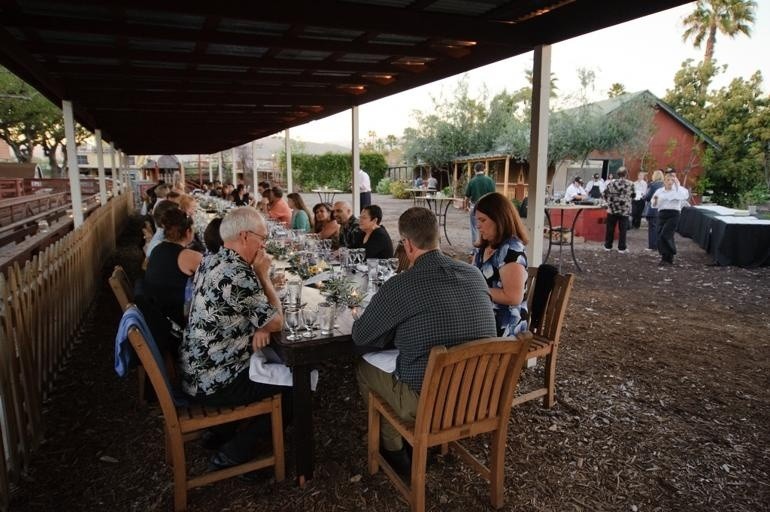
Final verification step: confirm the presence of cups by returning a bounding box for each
[268,266,302,308]
[318,302,335,335]
[367,258,378,281]
[323,238,332,250]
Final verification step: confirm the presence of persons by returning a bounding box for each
[563,165,689,266]
[333,200,365,248]
[202,179,313,231]
[470,191,532,338]
[415,175,423,189]
[358,204,394,258]
[306,202,341,249]
[360,166,371,207]
[177,205,318,482]
[140,160,198,264]
[187,217,224,321]
[144,207,204,327]
[427,174,438,201]
[351,207,499,471]
[464,162,496,255]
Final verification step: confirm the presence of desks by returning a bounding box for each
[677,203,770,268]
[309,189,345,205]
[404,188,437,206]
[415,197,459,246]
[525,204,601,272]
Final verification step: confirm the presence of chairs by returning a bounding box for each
[123,302,286,511]
[142,228,154,243]
[109,265,180,408]
[509,267,575,409]
[366,332,535,512]
[146,221,156,234]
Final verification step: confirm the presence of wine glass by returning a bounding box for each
[348,248,366,266]
[285,306,317,341]
[376,258,400,279]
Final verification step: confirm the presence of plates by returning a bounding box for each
[734,210,749,217]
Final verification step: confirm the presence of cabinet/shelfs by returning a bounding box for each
[0,176,100,204]
